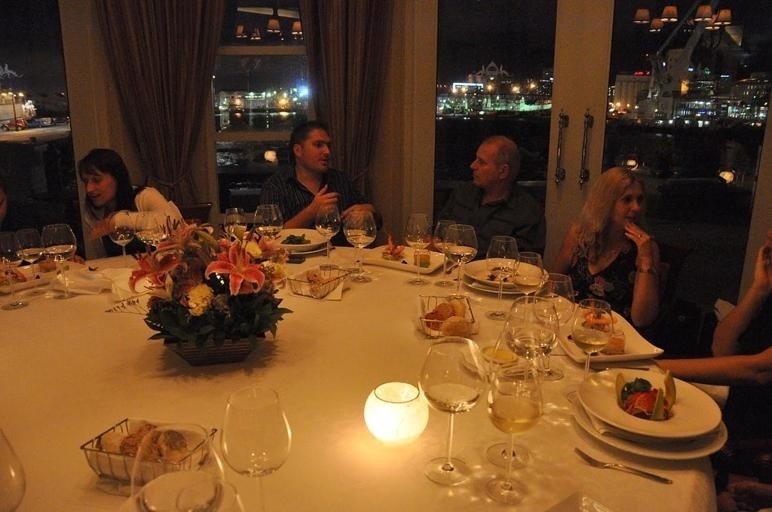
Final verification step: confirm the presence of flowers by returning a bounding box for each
[103,217,292,349]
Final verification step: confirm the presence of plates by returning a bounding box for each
[577,367,722,444]
[572,398,728,461]
[284,247,335,257]
[363,245,449,275]
[80,417,218,485]
[464,258,550,288]
[462,280,538,295]
[0,259,89,295]
[270,229,331,252]
[555,304,664,363]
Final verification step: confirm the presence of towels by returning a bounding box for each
[98,416,213,495]
[409,295,482,337]
[98,267,152,300]
[285,264,343,301]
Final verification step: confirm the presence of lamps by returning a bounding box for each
[633,0,733,34]
[235,5,306,49]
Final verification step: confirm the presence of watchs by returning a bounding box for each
[634,265,658,276]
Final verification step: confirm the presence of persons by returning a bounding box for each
[555,166,662,330]
[717,479,772,511]
[651,228,771,465]
[259,120,383,246]
[433,135,546,268]
[77,147,188,257]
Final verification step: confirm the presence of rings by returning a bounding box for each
[638,232,644,239]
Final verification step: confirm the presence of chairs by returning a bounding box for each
[173,203,213,226]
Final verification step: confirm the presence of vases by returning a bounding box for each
[164,330,266,366]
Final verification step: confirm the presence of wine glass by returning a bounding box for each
[253,204,283,264]
[0,427,27,512]
[485,363,544,505]
[433,219,459,287]
[443,224,479,302]
[108,210,135,268]
[0,232,29,311]
[224,208,248,240]
[343,211,376,283]
[532,273,575,381]
[40,224,77,298]
[504,296,559,359]
[14,227,47,297]
[315,203,340,263]
[565,299,613,403]
[512,252,543,324]
[130,421,224,512]
[405,214,434,286]
[486,236,515,321]
[418,336,486,489]
[137,211,169,248]
[486,333,539,468]
[219,386,291,511]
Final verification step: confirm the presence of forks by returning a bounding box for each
[574,446,673,485]
